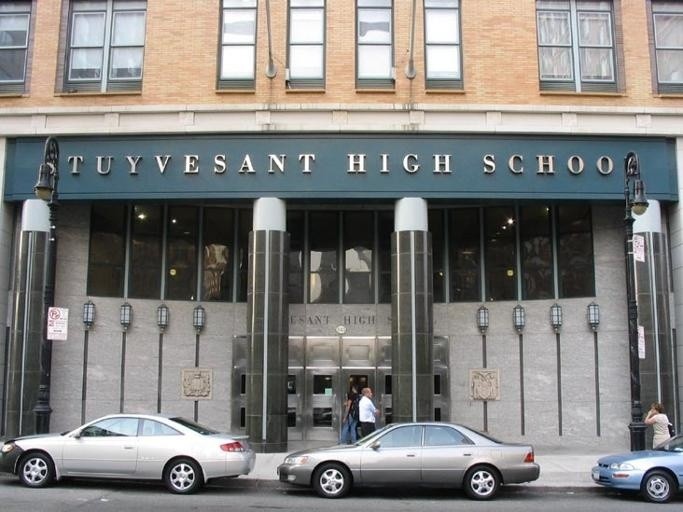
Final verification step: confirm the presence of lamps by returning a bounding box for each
[82,301,95,326]
[192,305,205,333]
[587,302,600,328]
[476,304,488,329]
[550,304,562,331]
[157,303,168,330]
[120,302,131,327]
[513,304,524,329]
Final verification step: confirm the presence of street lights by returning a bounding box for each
[30,135,60,435]
[625,149,648,452]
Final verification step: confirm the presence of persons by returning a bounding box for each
[358,386,380,438]
[643,399,675,454]
[342,392,359,445]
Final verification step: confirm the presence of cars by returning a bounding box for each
[591,434,682,503]
[0,411,257,495]
[276,421,539,499]
